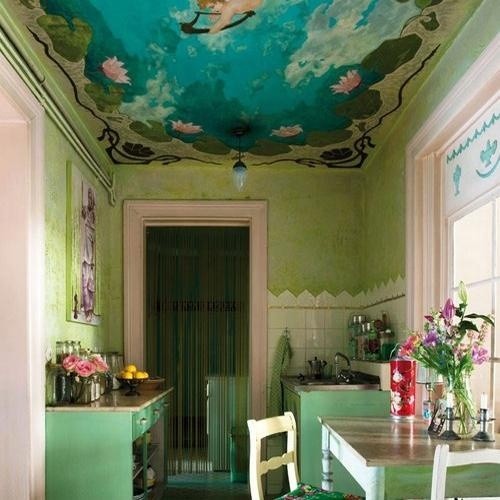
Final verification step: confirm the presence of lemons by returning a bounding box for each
[118,364,149,379]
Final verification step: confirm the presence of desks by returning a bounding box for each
[316,415,500,499]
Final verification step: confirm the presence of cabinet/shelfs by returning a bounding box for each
[203,373,249,471]
[282,384,391,497]
[44,395,169,500]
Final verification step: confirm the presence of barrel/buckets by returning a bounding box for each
[147,464,157,488]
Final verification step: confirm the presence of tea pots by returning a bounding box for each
[308,357,327,378]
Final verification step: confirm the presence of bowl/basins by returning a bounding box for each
[140,380,165,390]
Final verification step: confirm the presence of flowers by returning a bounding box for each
[395,279,496,435]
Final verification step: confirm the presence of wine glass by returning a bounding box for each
[115,376,149,396]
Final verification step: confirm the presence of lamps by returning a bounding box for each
[230,125,254,192]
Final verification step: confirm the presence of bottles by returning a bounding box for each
[351,316,393,363]
[46,340,123,406]
[146,465,154,488]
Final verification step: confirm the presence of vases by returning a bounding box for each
[429,374,483,441]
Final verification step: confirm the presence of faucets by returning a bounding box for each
[336,371,352,384]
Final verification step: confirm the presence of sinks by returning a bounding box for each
[304,380,340,386]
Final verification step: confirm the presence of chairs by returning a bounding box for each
[246,411,366,499]
[431,443,500,499]
